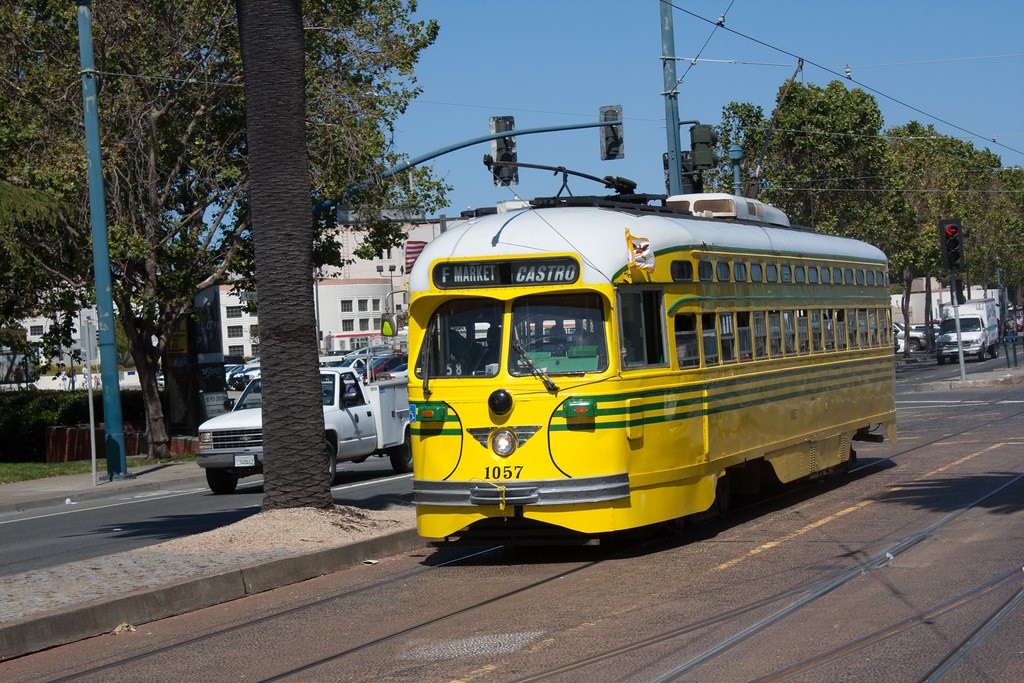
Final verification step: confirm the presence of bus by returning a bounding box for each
[0,346,41,393]
[380,194,898,553]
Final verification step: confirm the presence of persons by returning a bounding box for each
[1004,316,1017,336]
[333,376,356,394]
[81,362,100,389]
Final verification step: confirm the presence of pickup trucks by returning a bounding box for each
[197,368,412,495]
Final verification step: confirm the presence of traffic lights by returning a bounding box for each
[939,219,966,272]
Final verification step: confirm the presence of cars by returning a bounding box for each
[225,345,407,392]
[913,324,941,343]
[1007,315,1024,332]
[155,372,165,390]
[894,322,926,350]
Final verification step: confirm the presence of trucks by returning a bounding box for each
[937,297,1000,364]
[119,370,144,393]
